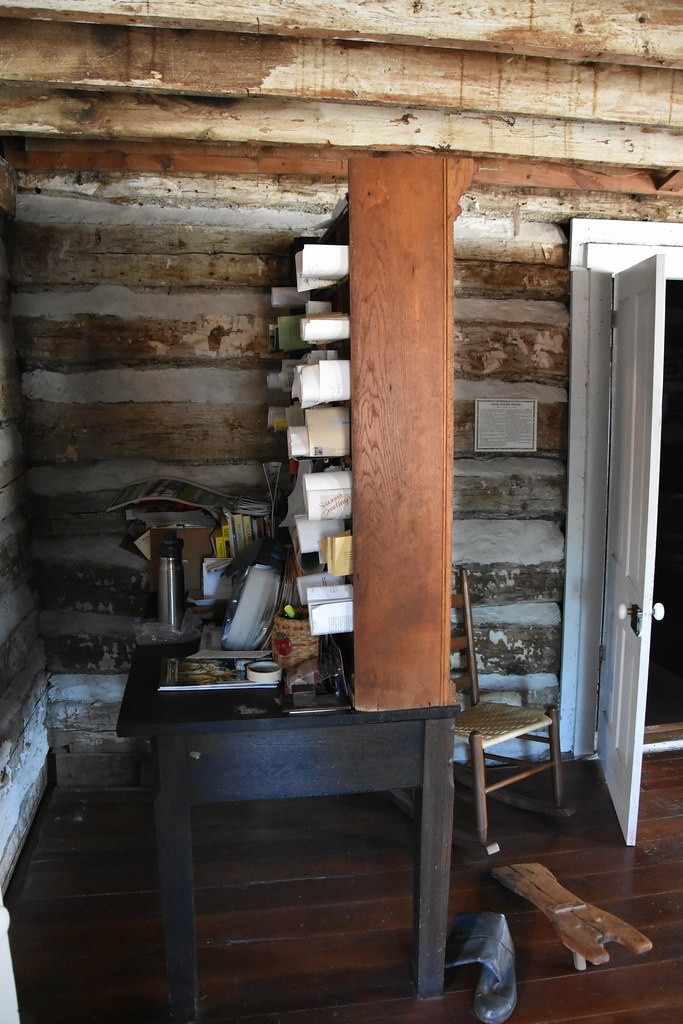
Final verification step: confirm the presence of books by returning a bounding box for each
[157,657,278,692]
[126,499,271,556]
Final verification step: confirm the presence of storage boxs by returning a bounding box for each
[137,526,213,593]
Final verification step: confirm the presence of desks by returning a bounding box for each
[114,642,461,1024]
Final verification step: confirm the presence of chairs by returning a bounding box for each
[448,569,575,857]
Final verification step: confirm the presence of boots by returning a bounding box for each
[444,912,518,1024]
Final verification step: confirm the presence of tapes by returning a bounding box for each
[246,662,282,682]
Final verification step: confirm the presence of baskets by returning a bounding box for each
[261,606,320,675]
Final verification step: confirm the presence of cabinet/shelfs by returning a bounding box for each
[290,153,461,710]
